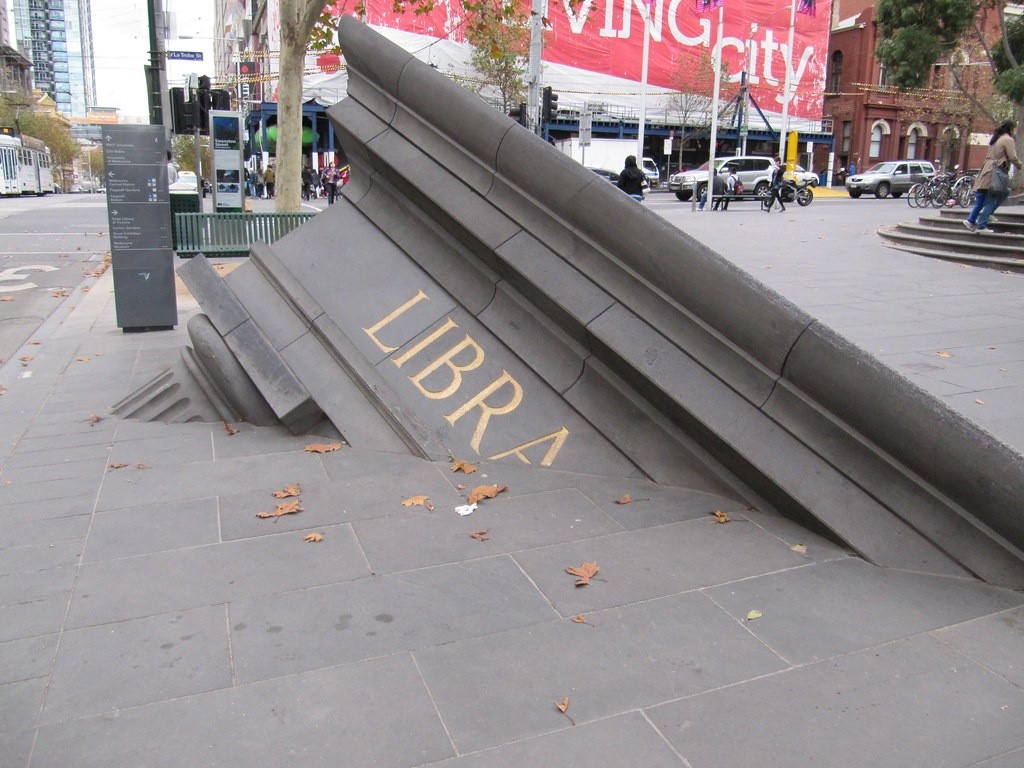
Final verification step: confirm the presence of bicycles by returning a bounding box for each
[907,164,980,208]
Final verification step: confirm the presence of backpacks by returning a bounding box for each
[731,173,744,195]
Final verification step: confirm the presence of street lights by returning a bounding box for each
[179,35,241,110]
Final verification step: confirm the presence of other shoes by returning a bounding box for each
[975,227,994,234]
[698,208,704,211]
[963,220,975,232]
[779,207,786,212]
[763,208,770,213]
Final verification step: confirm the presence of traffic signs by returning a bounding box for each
[100,124,178,328]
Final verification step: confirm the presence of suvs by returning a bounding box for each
[845,160,936,199]
[668,155,777,202]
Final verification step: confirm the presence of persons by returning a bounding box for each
[962,119,1023,235]
[165,151,177,185]
[763,157,786,213]
[618,155,645,203]
[246,161,352,206]
[696,168,724,211]
[849,161,855,176]
[713,167,740,211]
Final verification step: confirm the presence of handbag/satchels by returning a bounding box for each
[991,163,1013,194]
[640,172,651,197]
[321,176,326,183]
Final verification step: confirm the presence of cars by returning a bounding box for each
[69,184,82,194]
[780,163,820,187]
[90,185,106,194]
[588,168,620,186]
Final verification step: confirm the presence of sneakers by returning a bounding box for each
[987,215,998,223]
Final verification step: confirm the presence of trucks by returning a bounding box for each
[553,136,660,189]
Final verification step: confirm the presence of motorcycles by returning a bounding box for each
[766,177,817,206]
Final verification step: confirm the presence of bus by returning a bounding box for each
[0,126,56,198]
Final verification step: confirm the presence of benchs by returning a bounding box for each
[712,194,773,212]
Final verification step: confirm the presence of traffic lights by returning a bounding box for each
[239,61,259,77]
